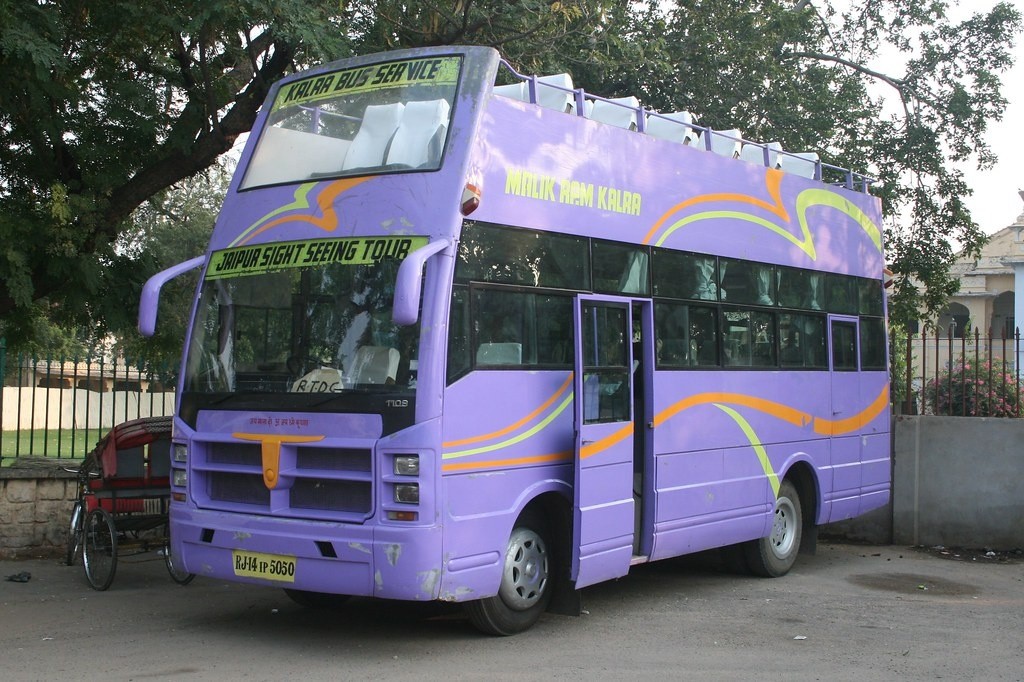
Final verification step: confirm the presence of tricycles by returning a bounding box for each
[56,416,196,592]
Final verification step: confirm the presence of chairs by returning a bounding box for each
[347,344,400,384]
[620,338,882,369]
[306,74,820,184]
[476,339,522,367]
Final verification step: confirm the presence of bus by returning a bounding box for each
[139,45,893,638]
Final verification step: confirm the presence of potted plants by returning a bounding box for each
[893,326,919,416]
